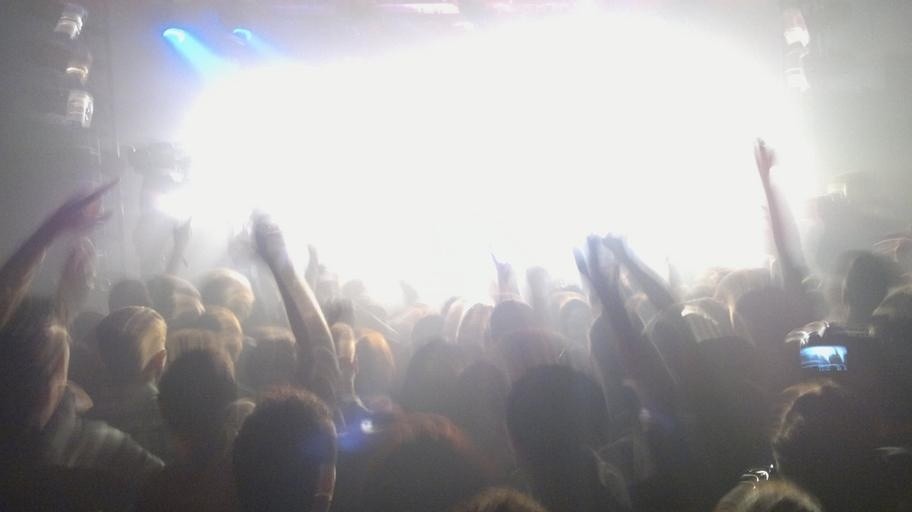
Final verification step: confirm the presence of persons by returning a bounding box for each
[0,141,911,512]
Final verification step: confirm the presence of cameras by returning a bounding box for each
[799,345,848,373]
